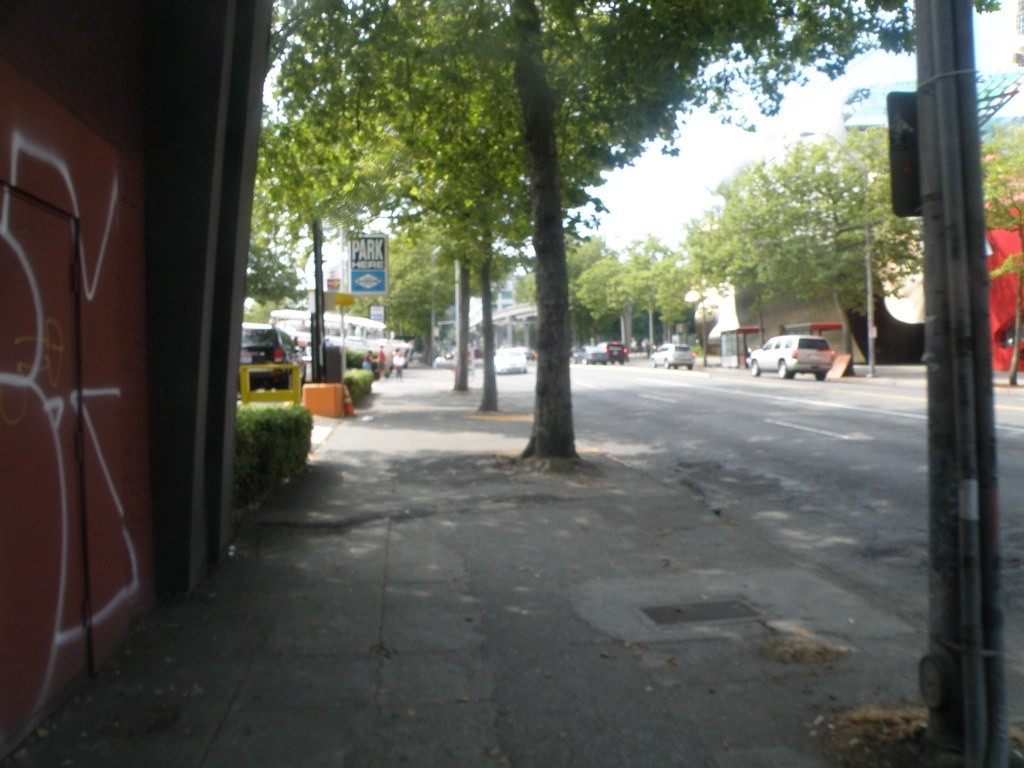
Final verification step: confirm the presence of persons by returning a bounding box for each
[362,350,374,370]
[377,345,386,370]
[392,349,405,378]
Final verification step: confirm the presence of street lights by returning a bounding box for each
[800,129,879,380]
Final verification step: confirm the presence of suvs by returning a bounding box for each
[239,323,307,395]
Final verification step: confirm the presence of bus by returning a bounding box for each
[267,310,392,378]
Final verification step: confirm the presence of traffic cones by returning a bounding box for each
[342,384,356,418]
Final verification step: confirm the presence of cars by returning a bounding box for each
[571,345,592,365]
[517,345,534,358]
[492,350,528,375]
[586,341,627,364]
[650,343,695,370]
[748,334,835,381]
